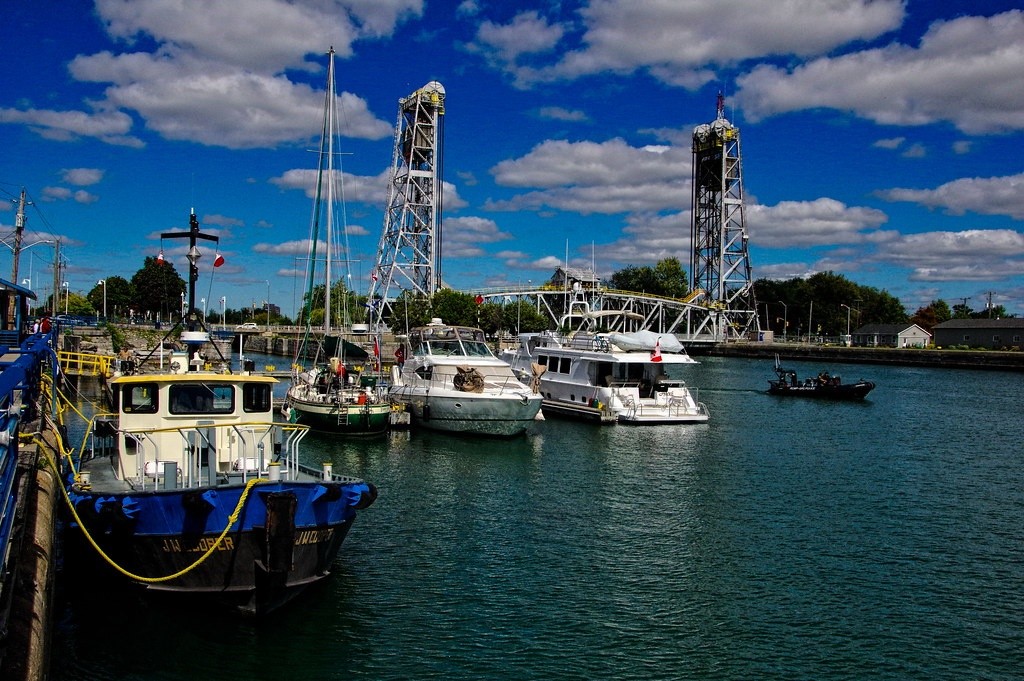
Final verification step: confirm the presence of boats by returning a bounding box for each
[511,282,710,426]
[767,368,876,404]
[65,202,377,618]
[388,317,544,438]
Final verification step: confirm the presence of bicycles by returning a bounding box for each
[591,332,609,354]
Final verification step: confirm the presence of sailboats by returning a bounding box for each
[284,44,395,441]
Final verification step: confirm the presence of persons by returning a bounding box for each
[817,372,830,386]
[118,347,132,361]
[32,316,52,334]
[394,343,407,367]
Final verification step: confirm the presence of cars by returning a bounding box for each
[237,323,258,330]
[57,315,84,325]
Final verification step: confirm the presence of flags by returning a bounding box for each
[651,340,662,362]
[374,336,379,357]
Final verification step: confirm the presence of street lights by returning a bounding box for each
[201,298,206,325]
[840,304,850,346]
[518,275,519,336]
[22,278,31,329]
[63,282,68,324]
[779,301,786,342]
[222,297,226,332]
[7,239,54,333]
[265,280,270,331]
[98,280,106,326]
[180,292,184,319]
